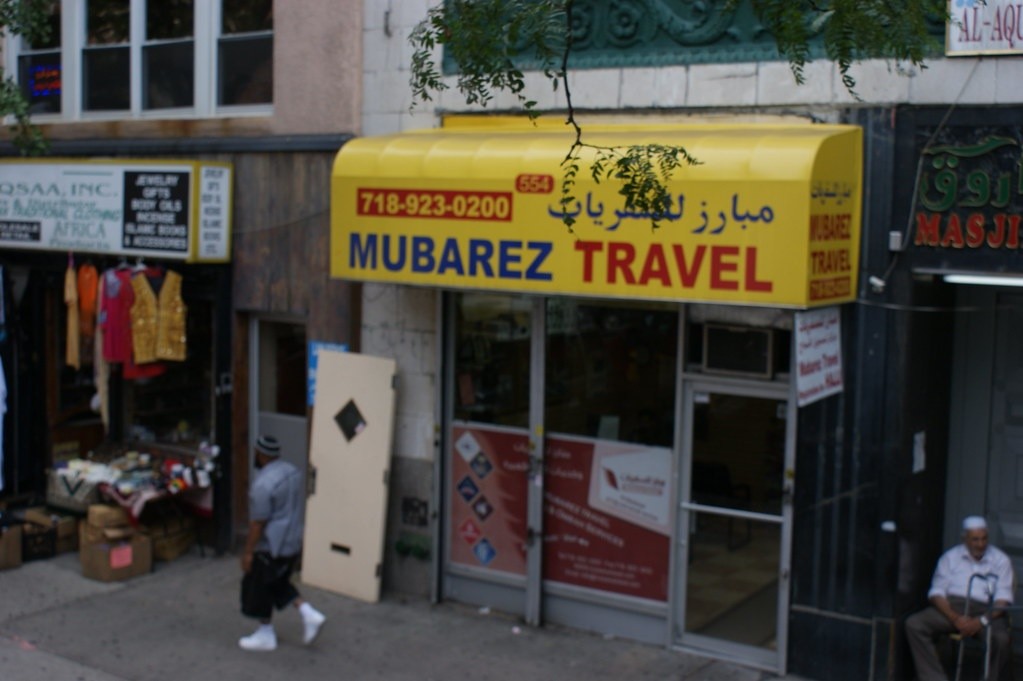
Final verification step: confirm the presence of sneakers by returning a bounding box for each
[298,602,326,646]
[239,626,278,652]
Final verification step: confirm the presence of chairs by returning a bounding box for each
[934,571,1020,679]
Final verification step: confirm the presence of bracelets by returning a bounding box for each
[979,615,989,626]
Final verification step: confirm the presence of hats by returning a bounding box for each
[962,515,987,530]
[254,433,281,457]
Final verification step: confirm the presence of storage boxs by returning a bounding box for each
[0,460,203,584]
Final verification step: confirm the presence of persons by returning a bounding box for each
[238,435,326,651]
[905,514,1014,681]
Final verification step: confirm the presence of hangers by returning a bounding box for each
[63,245,167,287]
